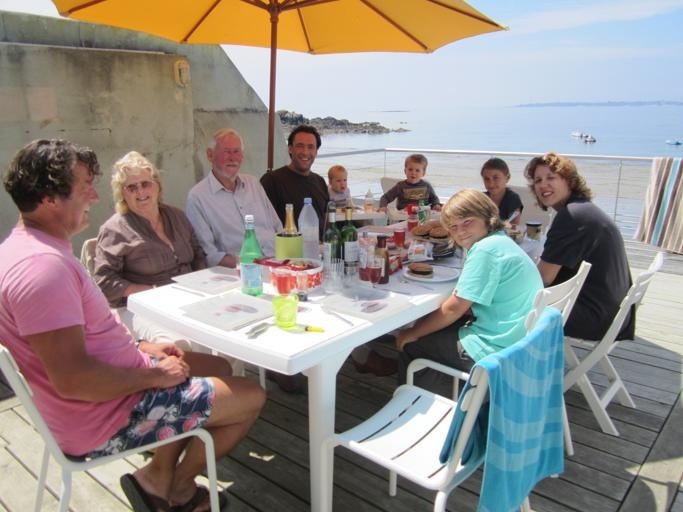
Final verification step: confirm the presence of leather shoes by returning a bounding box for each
[356,349,398,376]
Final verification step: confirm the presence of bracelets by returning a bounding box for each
[151,283,157,289]
[134,339,147,349]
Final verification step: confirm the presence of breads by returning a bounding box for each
[409,262,432,274]
[410,219,449,239]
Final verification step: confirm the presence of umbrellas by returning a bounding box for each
[49,1,514,171]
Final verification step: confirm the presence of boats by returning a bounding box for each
[584,134,596,143]
[665,139,682,146]
[570,131,587,139]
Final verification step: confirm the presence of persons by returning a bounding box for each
[376,154,442,215]
[323,164,365,232]
[258,125,399,377]
[522,153,637,342]
[393,188,547,402]
[478,157,524,239]
[0,138,268,511]
[91,151,209,311]
[185,127,330,397]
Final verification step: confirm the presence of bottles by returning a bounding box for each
[297,197,321,260]
[418,199,426,224]
[322,200,342,260]
[364,188,374,216]
[358,230,372,281]
[374,233,389,284]
[341,207,358,270]
[283,202,297,236]
[406,195,418,218]
[238,213,264,297]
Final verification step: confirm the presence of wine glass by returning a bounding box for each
[367,255,383,300]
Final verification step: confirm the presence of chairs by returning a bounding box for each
[563,252,663,437]
[380,178,409,225]
[0,344,221,512]
[334,308,564,512]
[80,239,245,378]
[406,260,592,456]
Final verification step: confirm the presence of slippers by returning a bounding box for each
[169,485,225,512]
[119,473,169,512]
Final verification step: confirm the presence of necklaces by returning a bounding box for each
[150,214,161,231]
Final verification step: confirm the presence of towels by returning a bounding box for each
[439,306,566,512]
[633,156,683,253]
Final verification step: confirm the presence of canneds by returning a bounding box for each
[527,222,542,240]
[408,220,418,231]
[394,231,405,247]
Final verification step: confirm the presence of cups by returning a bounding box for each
[272,293,301,328]
[295,272,309,301]
[319,256,360,293]
[407,219,418,231]
[273,233,304,259]
[270,265,293,295]
[393,229,406,248]
[526,220,543,241]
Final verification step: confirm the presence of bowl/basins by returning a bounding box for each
[268,258,323,293]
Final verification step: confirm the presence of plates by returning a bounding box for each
[402,265,459,281]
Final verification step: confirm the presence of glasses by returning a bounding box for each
[126,182,153,190]
